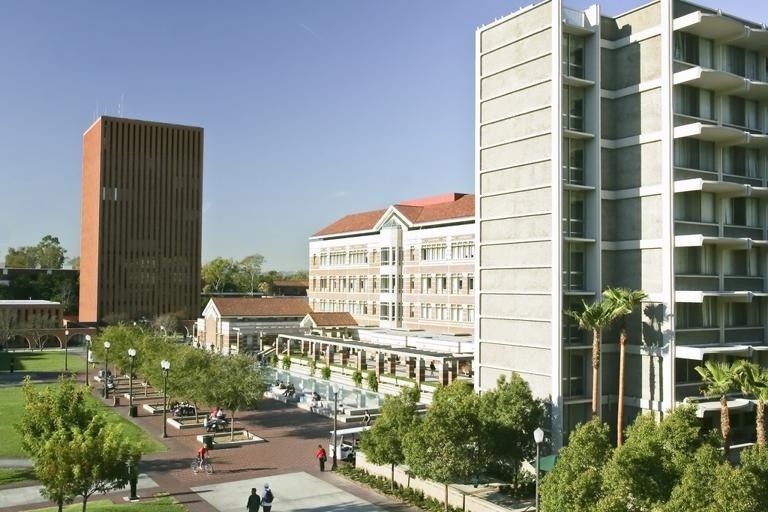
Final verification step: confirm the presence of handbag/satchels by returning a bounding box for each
[323,456,326,461]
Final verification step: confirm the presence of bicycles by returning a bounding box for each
[205,421,218,433]
[191,456,214,474]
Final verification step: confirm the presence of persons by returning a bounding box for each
[312,391,321,401]
[216,407,225,419]
[260,484,274,511]
[282,382,290,397]
[204,414,212,432]
[362,410,371,425]
[430,361,437,376]
[196,443,208,470]
[289,383,295,398]
[279,381,286,389]
[246,487,261,511]
[315,444,327,471]
[211,406,220,417]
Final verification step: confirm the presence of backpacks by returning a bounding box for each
[265,489,273,503]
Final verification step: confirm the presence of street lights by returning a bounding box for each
[128,348,136,416]
[64,330,69,369]
[103,342,110,399]
[85,335,91,386]
[161,360,170,438]
[534,428,544,512]
[333,385,339,468]
